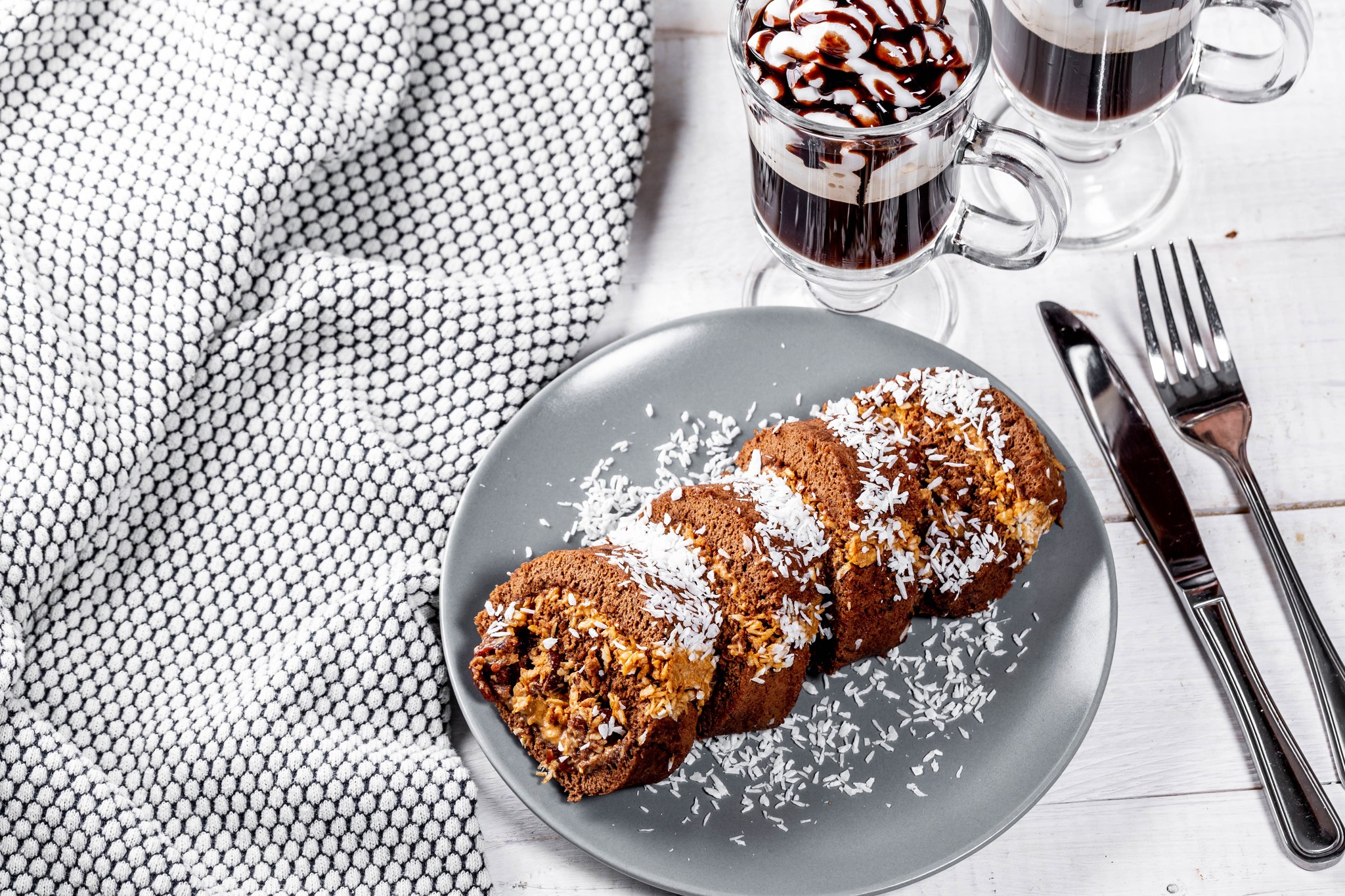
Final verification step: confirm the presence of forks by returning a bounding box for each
[1131,237,1345,791]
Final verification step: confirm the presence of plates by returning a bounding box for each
[440,307,1118,896]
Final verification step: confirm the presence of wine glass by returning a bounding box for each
[725,0,1072,348]
[974,0,1314,249]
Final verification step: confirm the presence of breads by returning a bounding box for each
[469,368,1067,803]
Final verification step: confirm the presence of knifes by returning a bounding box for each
[1036,301,1345,863]
